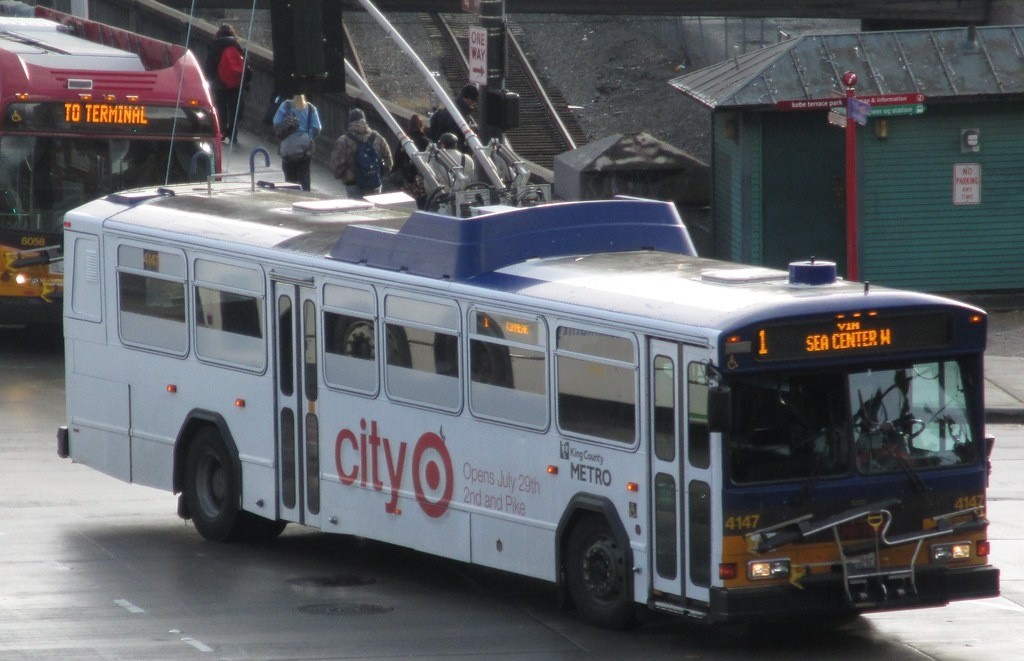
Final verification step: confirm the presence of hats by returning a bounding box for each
[349,108,365,121]
[461,85,479,101]
[438,133,458,144]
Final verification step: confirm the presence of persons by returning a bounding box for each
[202,23,252,145]
[694,376,937,484]
[393,114,435,202]
[428,82,477,163]
[426,130,474,208]
[329,108,394,199]
[1,114,178,233]
[272,82,322,192]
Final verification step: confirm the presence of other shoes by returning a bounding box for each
[220,130,229,141]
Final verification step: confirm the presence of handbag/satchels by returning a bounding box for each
[279,129,315,161]
[389,164,410,185]
[275,100,300,137]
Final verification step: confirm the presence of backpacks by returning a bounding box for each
[218,45,246,87]
[345,131,384,189]
[430,108,447,135]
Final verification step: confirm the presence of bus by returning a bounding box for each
[0,6,220,339]
[52,22,1000,637]
